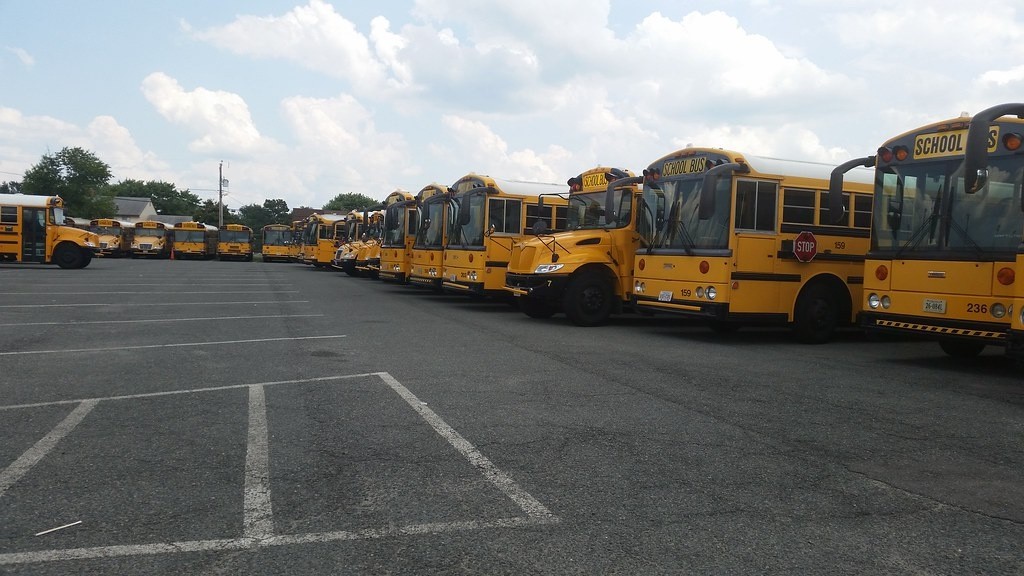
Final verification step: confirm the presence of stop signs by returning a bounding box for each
[793,231,817,263]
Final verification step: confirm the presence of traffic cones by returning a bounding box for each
[170,248,175,260]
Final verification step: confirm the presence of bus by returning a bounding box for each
[828,102,1024,369]
[89,217,135,258]
[0,193,101,269]
[259,224,293,263]
[42,216,91,232]
[602,144,1021,345]
[215,223,254,261]
[375,174,570,300]
[500,167,683,328]
[130,219,174,259]
[171,220,218,260]
[291,208,385,280]
